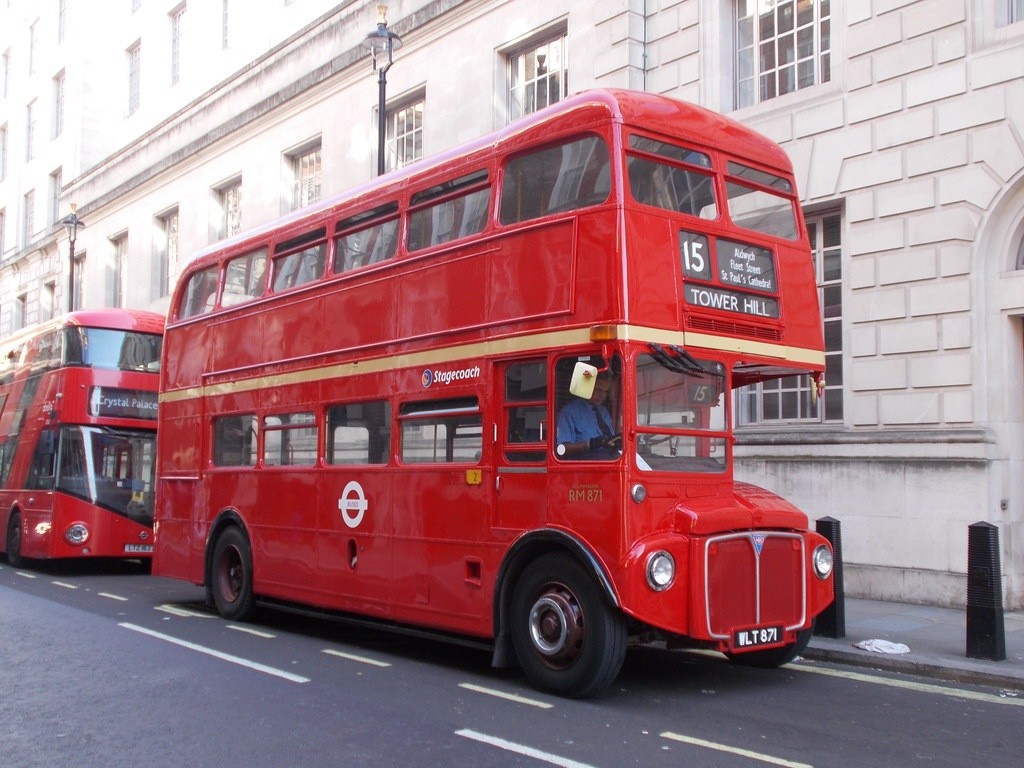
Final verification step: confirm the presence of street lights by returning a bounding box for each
[363,1,403,178]
[62,203,85,312]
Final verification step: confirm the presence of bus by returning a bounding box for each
[0,309,167,567]
[148,87,836,700]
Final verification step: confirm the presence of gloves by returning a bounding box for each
[590,437,614,453]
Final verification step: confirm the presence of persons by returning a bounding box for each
[555,363,622,461]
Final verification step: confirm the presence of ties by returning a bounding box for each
[595,407,613,438]
